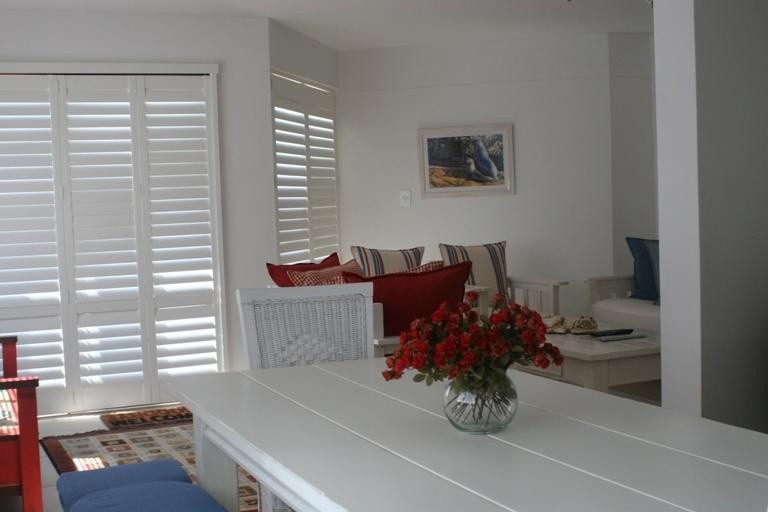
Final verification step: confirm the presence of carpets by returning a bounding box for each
[39,405,259,512]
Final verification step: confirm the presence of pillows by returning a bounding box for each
[625,237,660,305]
[264,239,508,335]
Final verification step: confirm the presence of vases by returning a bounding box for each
[444,367,517,433]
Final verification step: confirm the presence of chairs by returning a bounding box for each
[374,274,570,356]
[235,281,374,367]
[584,273,661,337]
[0,335,42,512]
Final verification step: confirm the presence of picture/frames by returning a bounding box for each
[416,121,516,199]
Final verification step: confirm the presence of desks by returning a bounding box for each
[157,353,768,512]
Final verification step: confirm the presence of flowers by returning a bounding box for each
[380,291,564,386]
